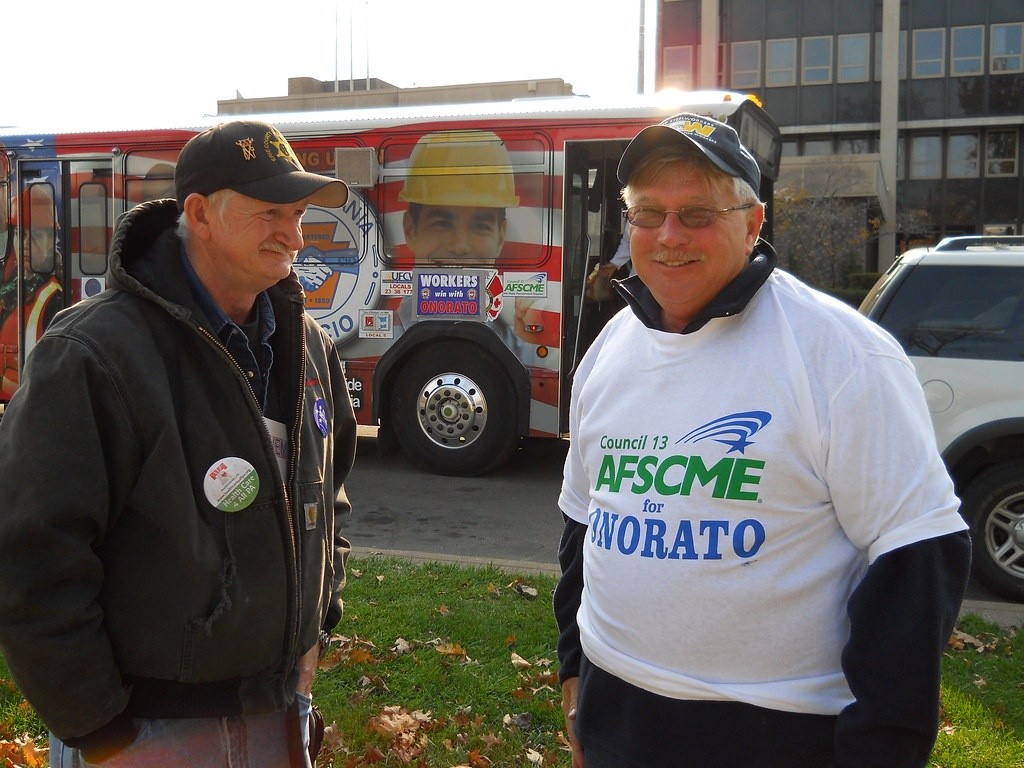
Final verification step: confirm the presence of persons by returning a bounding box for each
[552,114,973,768]
[600,218,637,277]
[398,129,550,366]
[141,163,177,201]
[0,121,359,768]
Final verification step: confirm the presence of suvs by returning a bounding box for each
[855,236,1024,605]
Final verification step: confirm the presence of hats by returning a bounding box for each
[617,114,762,196]
[175,118,348,214]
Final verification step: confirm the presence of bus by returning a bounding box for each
[0,90,785,480]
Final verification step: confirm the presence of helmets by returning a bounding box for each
[399,129,523,210]
[5,186,60,229]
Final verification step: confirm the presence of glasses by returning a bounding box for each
[620,199,756,229]
